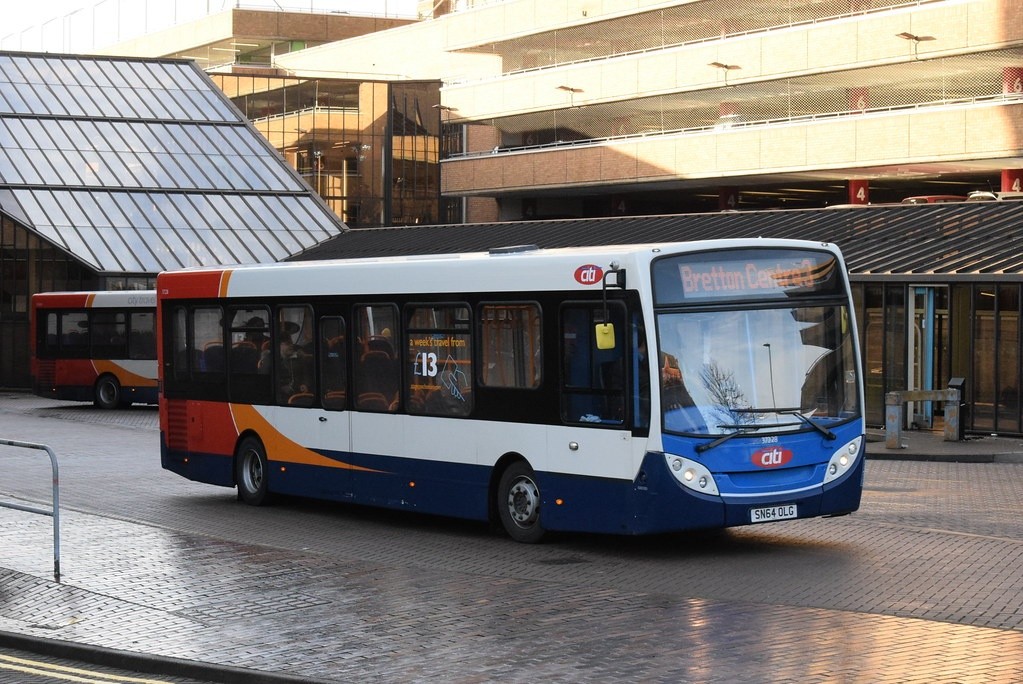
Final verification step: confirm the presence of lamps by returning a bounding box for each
[896,33,918,40]
[556,86,574,92]
[431,104,451,111]
[707,62,727,68]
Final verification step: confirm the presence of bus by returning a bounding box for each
[157,236,867,543]
[30,290,159,410]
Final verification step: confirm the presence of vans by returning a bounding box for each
[901,190,1023,204]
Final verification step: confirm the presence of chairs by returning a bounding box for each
[183,336,470,414]
[48,331,155,357]
[598,357,615,411]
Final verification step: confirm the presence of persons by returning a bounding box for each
[238,315,372,404]
[616,330,679,393]
[485,331,541,384]
